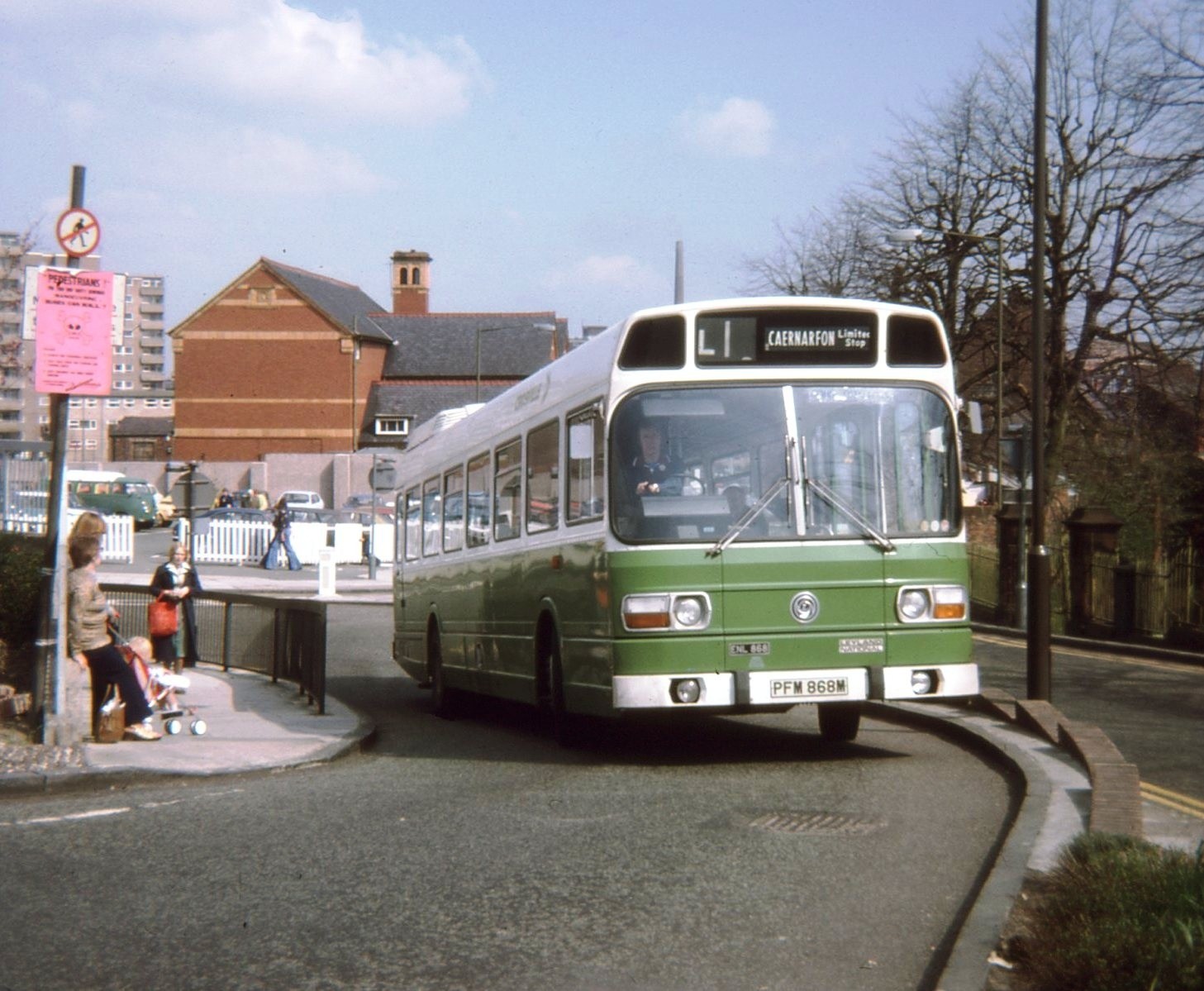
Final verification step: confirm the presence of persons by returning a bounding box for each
[66,512,160,739]
[262,503,302,570]
[148,540,201,673]
[219,487,234,511]
[615,424,687,516]
[242,487,261,508]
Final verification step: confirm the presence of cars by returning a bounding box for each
[263,494,395,533]
[172,508,273,542]
[276,490,325,511]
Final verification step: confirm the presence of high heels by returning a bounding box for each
[123,725,161,741]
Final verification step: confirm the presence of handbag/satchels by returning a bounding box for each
[97,682,126,743]
[148,591,178,636]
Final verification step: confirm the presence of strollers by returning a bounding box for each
[106,612,208,736]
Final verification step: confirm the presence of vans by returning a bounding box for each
[12,490,85,509]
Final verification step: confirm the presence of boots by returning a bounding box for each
[174,657,185,694]
[163,661,171,674]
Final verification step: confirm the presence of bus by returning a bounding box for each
[371,295,987,745]
[37,469,158,530]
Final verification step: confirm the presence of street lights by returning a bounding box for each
[476,322,556,403]
[885,225,1005,515]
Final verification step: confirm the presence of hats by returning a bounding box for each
[274,498,287,509]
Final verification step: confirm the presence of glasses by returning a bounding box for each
[95,548,103,552]
